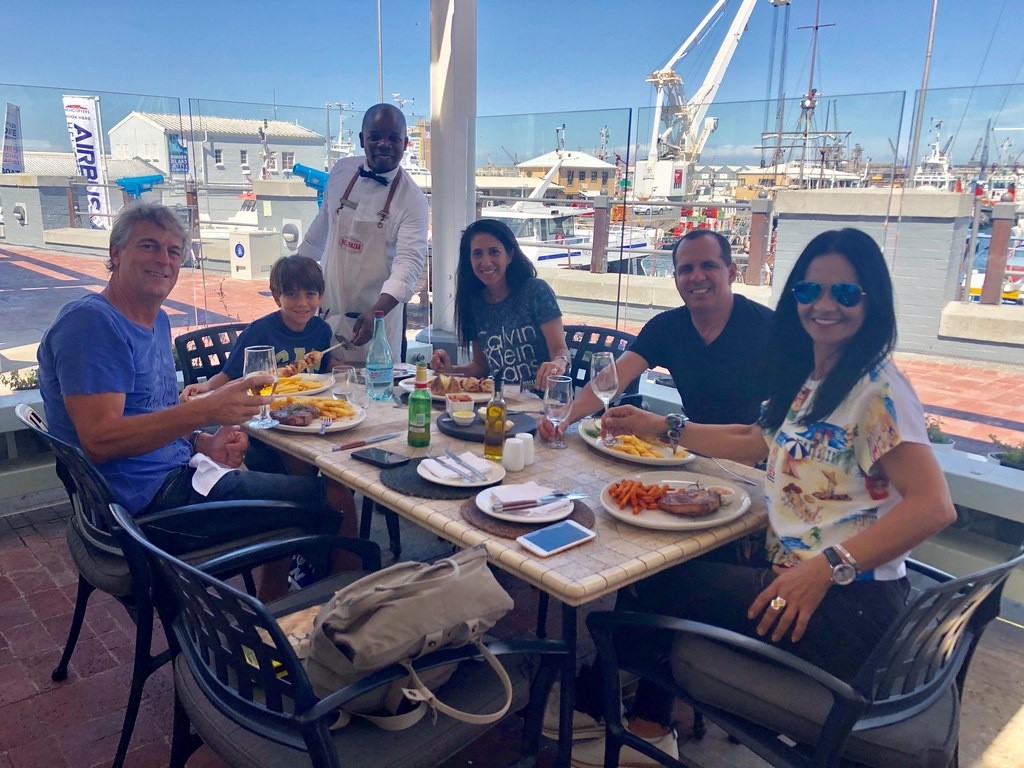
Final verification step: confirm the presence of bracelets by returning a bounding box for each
[189,430,203,455]
[834,543,862,572]
[659,412,689,444]
[554,355,572,369]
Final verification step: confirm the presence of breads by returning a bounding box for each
[462,376,494,392]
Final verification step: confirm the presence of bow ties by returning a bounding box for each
[359,166,389,187]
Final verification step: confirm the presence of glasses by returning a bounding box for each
[792,280,866,309]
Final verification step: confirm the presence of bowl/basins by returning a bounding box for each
[444,392,489,424]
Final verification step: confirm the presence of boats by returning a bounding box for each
[324,1,1024,269]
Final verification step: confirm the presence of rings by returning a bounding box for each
[550,369,557,374]
[243,455,245,459]
[771,595,786,610]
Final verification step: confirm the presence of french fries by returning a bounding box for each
[259,377,323,395]
[268,396,357,420]
[608,433,687,459]
[609,478,670,513]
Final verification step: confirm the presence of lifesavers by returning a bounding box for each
[555,232,565,244]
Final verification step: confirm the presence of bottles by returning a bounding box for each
[407,363,432,448]
[482,369,507,460]
[364,311,395,402]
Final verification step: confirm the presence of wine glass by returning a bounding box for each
[590,351,624,451]
[542,375,574,450]
[242,345,280,429]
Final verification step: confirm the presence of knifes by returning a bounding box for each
[425,447,489,483]
[489,490,594,513]
[329,431,403,452]
[508,406,547,417]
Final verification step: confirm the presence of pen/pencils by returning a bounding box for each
[318,307,322,318]
[323,307,331,321]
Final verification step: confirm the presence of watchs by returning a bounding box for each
[823,546,856,586]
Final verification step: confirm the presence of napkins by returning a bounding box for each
[421,452,491,480]
[493,481,571,516]
[189,452,249,496]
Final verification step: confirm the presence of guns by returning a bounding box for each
[292,162,330,208]
[116,174,164,202]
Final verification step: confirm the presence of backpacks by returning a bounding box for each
[298,541,513,731]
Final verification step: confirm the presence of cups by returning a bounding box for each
[345,369,373,408]
[501,432,535,472]
[331,365,355,403]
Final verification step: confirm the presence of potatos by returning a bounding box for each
[431,373,461,396]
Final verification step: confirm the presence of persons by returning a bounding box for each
[430,218,571,398]
[540,228,959,768]
[37,202,361,606]
[178,255,332,404]
[295,103,429,364]
[536,228,776,471]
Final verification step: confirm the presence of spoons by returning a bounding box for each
[684,449,757,485]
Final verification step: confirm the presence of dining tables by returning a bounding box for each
[241,363,769,768]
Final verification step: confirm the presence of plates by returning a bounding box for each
[397,370,508,402]
[600,469,757,533]
[578,415,708,466]
[238,364,377,435]
[475,482,585,524]
[362,360,414,380]
[418,449,509,487]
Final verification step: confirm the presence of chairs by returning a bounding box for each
[14,323,1024,768]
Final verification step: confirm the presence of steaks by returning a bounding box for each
[656,488,721,515]
[269,404,320,426]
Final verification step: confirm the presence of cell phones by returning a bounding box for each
[351,447,410,468]
[517,519,597,557]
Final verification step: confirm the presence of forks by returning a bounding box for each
[318,416,335,437]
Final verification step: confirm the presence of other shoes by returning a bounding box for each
[541,703,628,740]
[570,725,679,768]
